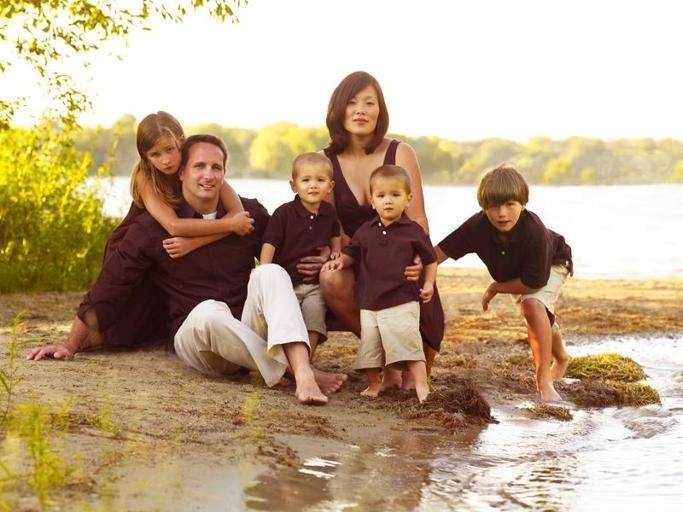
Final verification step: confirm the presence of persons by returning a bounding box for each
[433,162,573,403]
[320,164,436,405]
[25,135,347,405]
[259,152,343,365]
[317,72,445,392]
[102,111,254,348]
